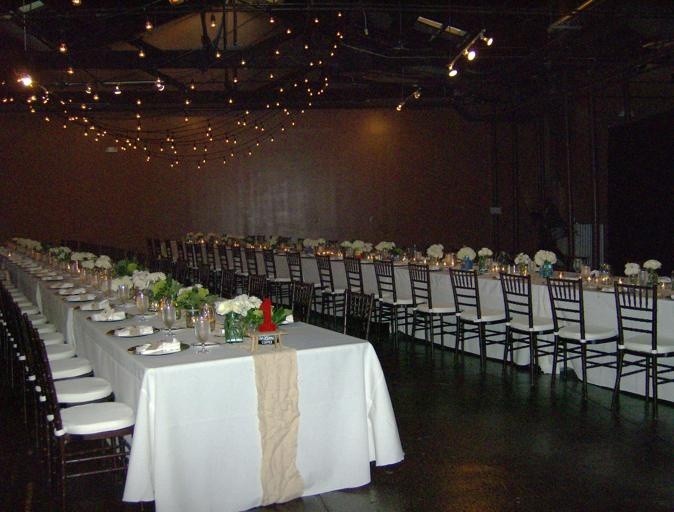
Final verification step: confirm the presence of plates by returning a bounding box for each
[0,248,97,301]
[85,308,129,323]
[106,324,159,338]
[127,339,191,355]
[72,300,115,314]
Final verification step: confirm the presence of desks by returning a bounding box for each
[188,231,673,402]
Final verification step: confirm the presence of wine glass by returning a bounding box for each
[4,241,111,299]
[382,248,670,299]
[134,291,151,323]
[194,318,209,355]
[161,304,177,336]
[116,285,132,314]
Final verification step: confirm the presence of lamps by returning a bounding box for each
[485,33,494,48]
[448,68,458,78]
[463,51,478,62]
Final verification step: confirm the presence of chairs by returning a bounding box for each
[1,264,134,510]
